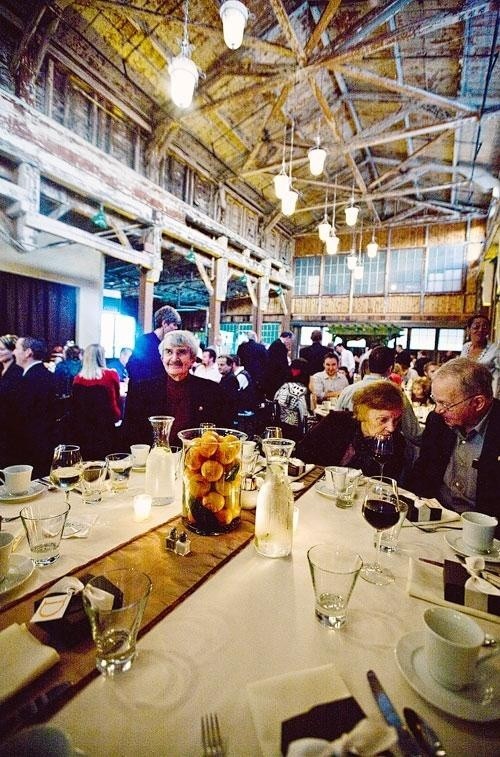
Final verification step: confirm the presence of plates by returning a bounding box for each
[314,481,335,498]
[396,630,499,722]
[0,554,37,594]
[447,529,500,565]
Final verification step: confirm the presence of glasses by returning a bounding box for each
[431,395,474,411]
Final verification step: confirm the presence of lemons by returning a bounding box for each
[184,432,242,526]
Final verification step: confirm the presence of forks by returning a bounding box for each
[199,709,228,756]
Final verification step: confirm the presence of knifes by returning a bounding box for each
[367,668,449,757]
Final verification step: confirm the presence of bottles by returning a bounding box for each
[166,527,191,556]
[263,426,284,437]
[143,413,178,504]
[201,422,216,432]
[254,436,297,556]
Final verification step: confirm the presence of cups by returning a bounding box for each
[1,727,84,757]
[308,544,364,633]
[177,427,248,536]
[0,444,179,582]
[423,608,487,693]
[374,499,409,554]
[461,509,498,555]
[79,568,157,677]
[323,466,361,509]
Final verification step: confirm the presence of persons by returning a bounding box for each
[461,314,500,399]
[123,305,184,397]
[405,356,500,540]
[1,330,456,484]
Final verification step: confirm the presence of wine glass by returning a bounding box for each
[362,430,394,476]
[361,476,400,587]
